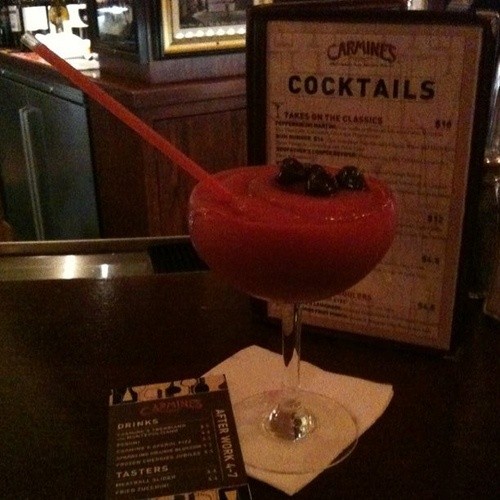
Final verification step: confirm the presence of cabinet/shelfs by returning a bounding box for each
[84,76,250,240]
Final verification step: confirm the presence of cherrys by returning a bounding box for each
[274,157,367,198]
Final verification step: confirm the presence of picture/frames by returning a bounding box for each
[133,0,275,86]
[243,5,496,359]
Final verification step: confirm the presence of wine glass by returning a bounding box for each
[188,162,399,474]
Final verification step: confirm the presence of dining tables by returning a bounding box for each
[0,266,500,500]
